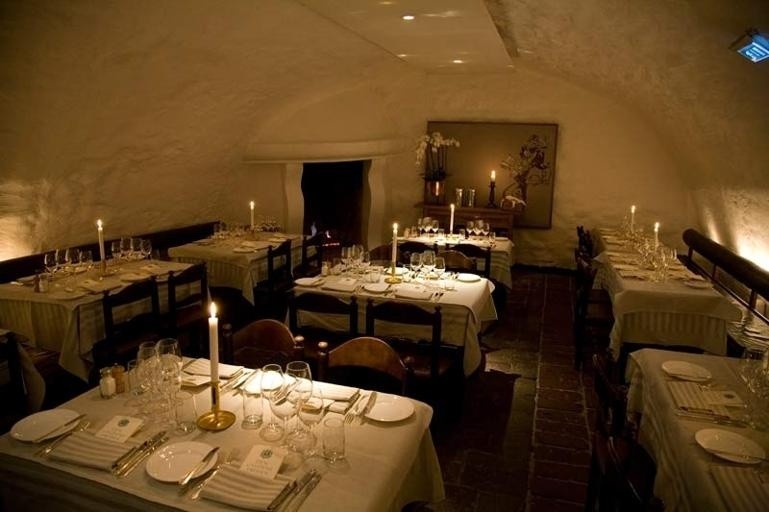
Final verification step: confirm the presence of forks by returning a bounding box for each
[191,447,239,501]
[342,394,368,425]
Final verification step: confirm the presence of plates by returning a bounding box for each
[9,408,78,443]
[684,279,714,290]
[355,392,416,423]
[694,426,765,466]
[494,236,509,241]
[662,358,712,382]
[456,272,481,283]
[241,371,297,389]
[294,278,394,294]
[145,441,217,483]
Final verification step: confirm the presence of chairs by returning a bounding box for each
[574,227,768,511]
[0,203,510,398]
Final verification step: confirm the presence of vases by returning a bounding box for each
[426,180,446,196]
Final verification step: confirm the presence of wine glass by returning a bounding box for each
[259,358,325,456]
[128,335,186,428]
[339,241,446,286]
[44,214,282,295]
[416,215,491,242]
[612,215,676,289]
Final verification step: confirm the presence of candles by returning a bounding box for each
[630,205,637,228]
[490,169,496,187]
[248,202,256,229]
[392,221,398,265]
[653,223,660,250]
[206,299,219,384]
[96,218,105,261]
[450,204,457,235]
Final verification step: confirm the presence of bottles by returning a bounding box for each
[98,361,127,399]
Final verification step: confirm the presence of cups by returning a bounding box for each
[489,231,495,244]
[401,226,417,239]
[323,417,346,463]
[240,383,263,425]
[169,390,200,432]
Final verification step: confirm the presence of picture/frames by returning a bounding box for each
[421,119,561,230]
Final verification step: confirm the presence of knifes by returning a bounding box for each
[266,467,322,512]
[114,430,168,476]
[28,414,85,443]
[359,392,378,423]
[176,444,220,487]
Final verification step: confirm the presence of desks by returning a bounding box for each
[1,356,433,512]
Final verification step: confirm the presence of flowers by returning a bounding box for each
[412,129,461,182]
